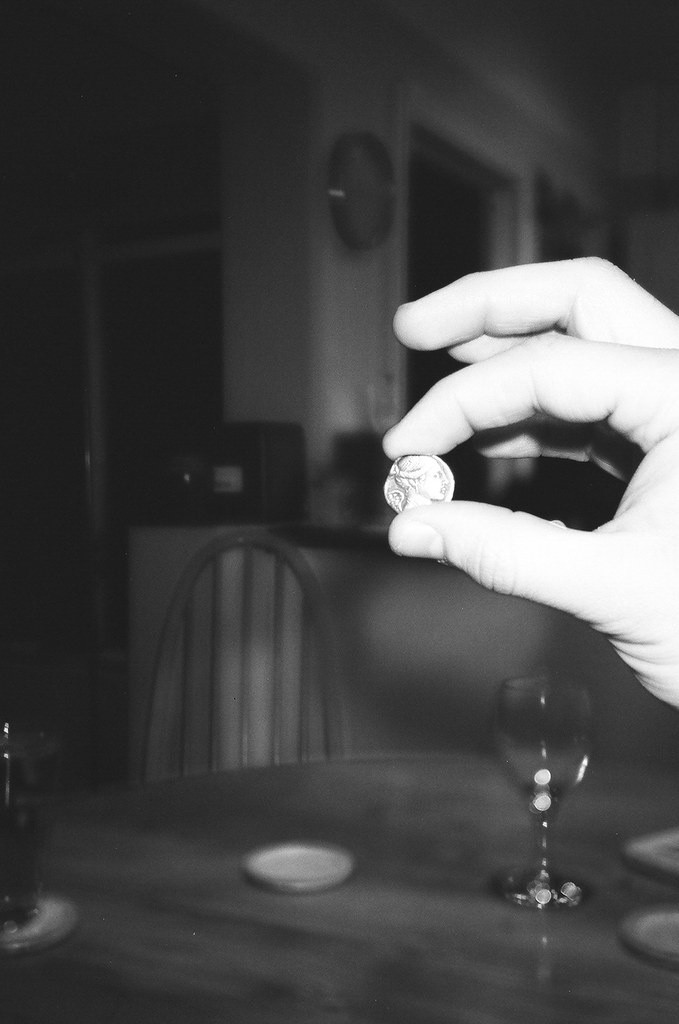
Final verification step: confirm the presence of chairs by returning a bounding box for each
[140,529,348,784]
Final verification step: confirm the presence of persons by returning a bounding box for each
[381,255,679,707]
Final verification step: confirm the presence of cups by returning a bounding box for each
[0,715,81,926]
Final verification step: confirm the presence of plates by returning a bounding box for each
[621,827,679,880]
[0,893,81,954]
[618,902,679,970]
[240,839,354,894]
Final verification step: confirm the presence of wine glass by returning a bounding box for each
[495,672,596,905]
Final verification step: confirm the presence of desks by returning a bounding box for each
[26,751,679,1024]
